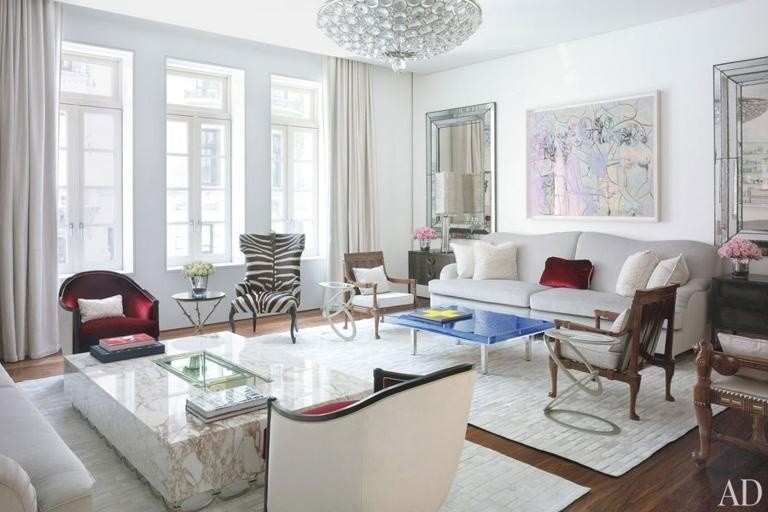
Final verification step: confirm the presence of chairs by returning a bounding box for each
[342,249,418,339]
[228,230,306,343]
[688,339,767,469]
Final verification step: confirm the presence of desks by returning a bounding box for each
[538,323,627,437]
[406,249,456,295]
[171,290,226,334]
[318,279,358,340]
[448,241,474,278]
[709,269,768,356]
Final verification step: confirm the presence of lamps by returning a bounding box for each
[462,172,484,229]
[434,171,463,254]
[311,0,484,73]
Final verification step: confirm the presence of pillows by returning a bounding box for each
[350,265,392,295]
[75,293,126,323]
[717,332,768,383]
[615,249,659,295]
[540,254,594,291]
[646,251,690,291]
[470,239,518,281]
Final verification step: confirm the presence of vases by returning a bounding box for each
[729,255,750,280]
[189,273,209,292]
[419,239,432,251]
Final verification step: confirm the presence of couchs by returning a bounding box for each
[0,348,96,512]
[58,267,160,354]
[426,230,716,360]
[261,360,478,510]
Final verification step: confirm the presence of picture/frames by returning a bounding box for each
[710,57,768,257]
[521,87,662,225]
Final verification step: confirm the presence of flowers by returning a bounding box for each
[183,260,215,278]
[411,226,437,240]
[717,235,764,261]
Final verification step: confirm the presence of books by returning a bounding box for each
[185,385,269,426]
[99,333,155,353]
[408,307,473,326]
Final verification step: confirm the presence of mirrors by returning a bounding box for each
[422,100,499,234]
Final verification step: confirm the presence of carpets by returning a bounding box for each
[11,328,593,512]
[251,311,736,477]
